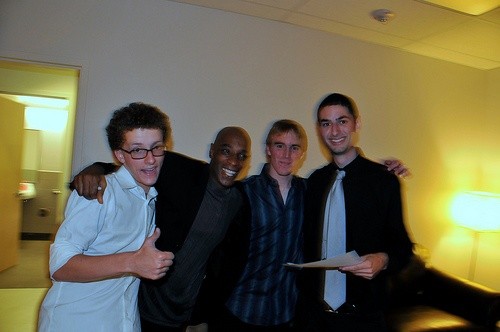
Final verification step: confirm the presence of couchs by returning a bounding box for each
[384,257,500,332]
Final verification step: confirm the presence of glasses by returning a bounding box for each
[117,144,168,159]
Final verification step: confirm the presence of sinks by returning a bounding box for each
[18,183,37,200]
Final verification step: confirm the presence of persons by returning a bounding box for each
[70,126,252,332]
[37,101,175,331]
[304,93,412,332]
[212,118,410,332]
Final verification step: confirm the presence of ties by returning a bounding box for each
[320,170,346,313]
